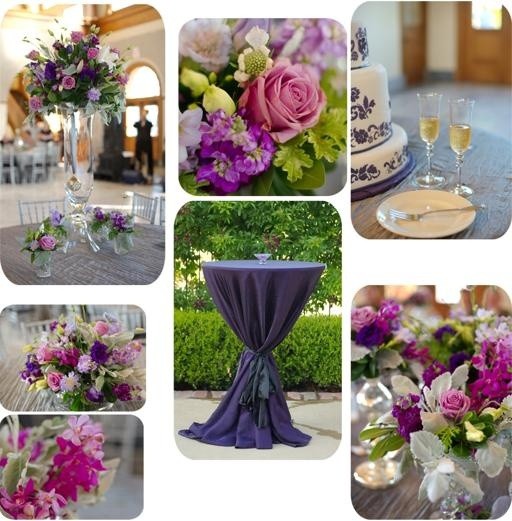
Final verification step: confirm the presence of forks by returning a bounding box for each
[389,204,486,222]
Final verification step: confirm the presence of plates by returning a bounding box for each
[375,189,477,239]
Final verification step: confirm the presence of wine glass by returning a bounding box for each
[253,253,274,264]
[446,96,474,197]
[415,93,445,188]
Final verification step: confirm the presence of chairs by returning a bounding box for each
[0,141,55,185]
[18,198,65,225]
[132,192,165,225]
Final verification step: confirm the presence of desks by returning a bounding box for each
[351,116,512,239]
[0,222,165,285]
[200,260,326,442]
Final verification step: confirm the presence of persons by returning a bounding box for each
[0,119,86,184]
[132,107,155,183]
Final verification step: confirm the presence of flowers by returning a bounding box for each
[178,18,347,195]
[92,206,136,240]
[350,297,512,520]
[0,415,111,520]
[17,309,144,411]
[13,11,137,126]
[17,208,68,264]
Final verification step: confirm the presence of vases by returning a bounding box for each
[59,108,102,254]
[36,250,51,278]
[113,232,134,256]
